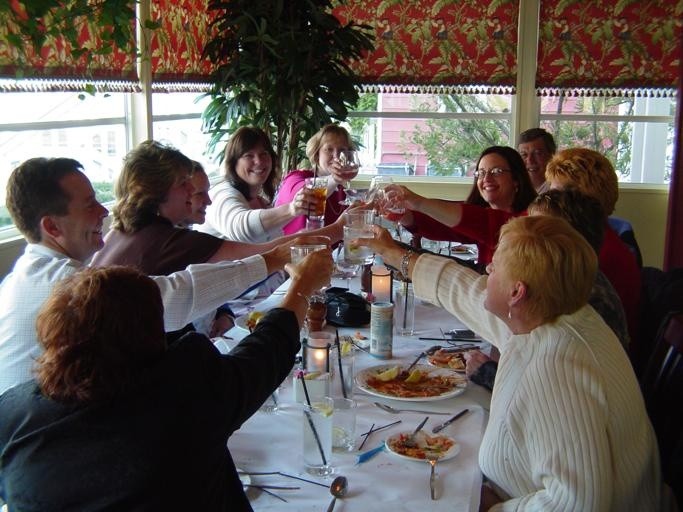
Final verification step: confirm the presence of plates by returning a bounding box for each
[384,429,470,462]
[426,348,466,372]
[234,468,252,494]
[352,361,469,403]
[233,314,251,332]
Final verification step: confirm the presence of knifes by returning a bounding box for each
[431,409,469,434]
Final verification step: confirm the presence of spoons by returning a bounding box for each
[427,344,480,353]
[369,401,452,416]
[326,476,349,512]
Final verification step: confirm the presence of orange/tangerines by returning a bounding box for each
[250,311,261,322]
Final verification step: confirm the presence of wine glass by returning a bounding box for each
[368,174,407,231]
[335,150,359,194]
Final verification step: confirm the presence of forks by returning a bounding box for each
[347,335,370,355]
[427,455,440,502]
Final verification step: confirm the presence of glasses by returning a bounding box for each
[474,168,511,177]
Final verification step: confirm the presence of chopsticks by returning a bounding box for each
[358,420,403,451]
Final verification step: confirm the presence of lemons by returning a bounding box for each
[375,366,399,381]
[304,372,320,380]
[312,402,333,418]
[341,341,351,357]
[405,369,421,382]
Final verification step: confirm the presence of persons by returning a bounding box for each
[381,148,642,351]
[0,158,333,391]
[0,248,334,512]
[174,160,238,338]
[192,126,318,300]
[377,146,538,266]
[382,188,633,356]
[86,139,379,340]
[516,127,555,194]
[350,216,662,512]
[273,124,362,249]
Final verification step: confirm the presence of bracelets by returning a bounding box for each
[283,290,312,308]
[400,249,416,281]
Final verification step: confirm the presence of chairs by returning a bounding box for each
[632,265,683,510]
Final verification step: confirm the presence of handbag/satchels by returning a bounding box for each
[325,285,373,328]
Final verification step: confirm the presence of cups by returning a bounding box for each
[303,176,329,232]
[301,394,358,470]
[305,331,337,374]
[258,387,281,415]
[288,243,332,288]
[342,208,377,266]
[393,287,418,337]
